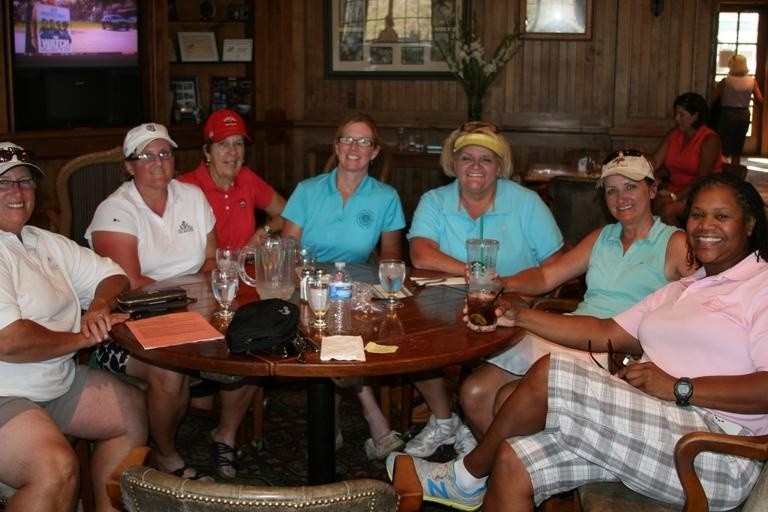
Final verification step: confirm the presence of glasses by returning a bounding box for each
[128,152,174,160]
[339,136,374,147]
[1,177,35,190]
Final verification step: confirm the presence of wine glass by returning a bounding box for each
[305,283,329,328]
[379,306,406,342]
[210,268,239,319]
[378,259,406,310]
[214,245,240,272]
[294,248,317,304]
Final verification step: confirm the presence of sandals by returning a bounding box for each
[163,459,214,482]
[209,432,240,481]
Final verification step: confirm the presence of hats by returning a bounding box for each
[596,150,656,189]
[731,56,751,75]
[204,109,251,143]
[0,141,48,181]
[454,134,503,161]
[122,123,178,156]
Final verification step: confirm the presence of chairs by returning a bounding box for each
[302,143,394,183]
[546,177,618,252]
[720,162,747,180]
[56,146,248,449]
[1,208,94,512]
[575,432,768,512]
[508,299,578,348]
[562,148,608,164]
[106,446,423,512]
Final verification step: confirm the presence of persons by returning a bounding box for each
[280,111,405,459]
[646,94,724,228]
[711,54,767,167]
[461,152,700,443]
[35,7,72,53]
[365,14,402,63]
[85,123,261,488]
[384,174,763,511]
[0,142,149,512]
[408,120,568,454]
[174,110,287,421]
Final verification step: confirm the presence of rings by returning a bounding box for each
[94,311,103,321]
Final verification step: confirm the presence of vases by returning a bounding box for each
[468,96,483,121]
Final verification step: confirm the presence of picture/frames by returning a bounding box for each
[169,74,199,123]
[176,30,221,63]
[516,0,596,42]
[321,0,469,81]
[221,35,254,64]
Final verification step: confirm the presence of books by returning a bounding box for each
[124,309,226,349]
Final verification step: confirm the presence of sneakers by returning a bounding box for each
[403,414,457,458]
[453,427,474,452]
[371,432,401,455]
[384,448,488,510]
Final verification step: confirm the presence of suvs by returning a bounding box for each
[102,15,130,31]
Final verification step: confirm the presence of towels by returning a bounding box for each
[319,332,366,362]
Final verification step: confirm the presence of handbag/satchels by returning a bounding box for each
[115,285,188,311]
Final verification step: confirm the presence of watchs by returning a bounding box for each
[673,376,693,407]
[261,222,272,239]
[669,191,680,206]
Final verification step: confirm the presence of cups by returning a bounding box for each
[467,289,498,332]
[465,239,500,292]
[237,234,296,301]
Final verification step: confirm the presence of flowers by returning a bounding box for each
[432,0,540,120]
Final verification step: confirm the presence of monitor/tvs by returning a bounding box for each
[9,1,141,68]
